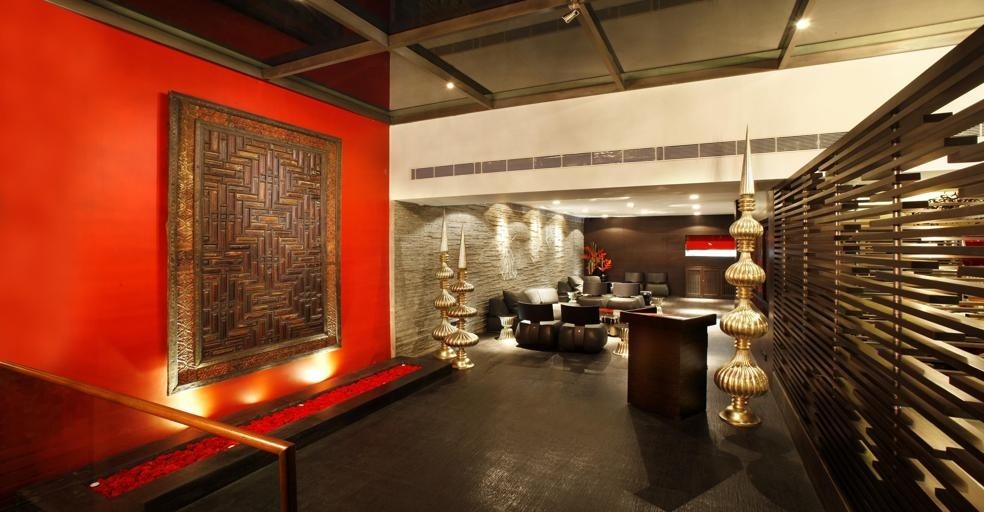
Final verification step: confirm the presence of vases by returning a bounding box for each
[599,270,608,281]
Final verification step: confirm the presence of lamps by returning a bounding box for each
[557,0,580,25]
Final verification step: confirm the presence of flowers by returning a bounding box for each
[580,241,613,275]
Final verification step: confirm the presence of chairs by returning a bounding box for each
[487,271,670,355]
